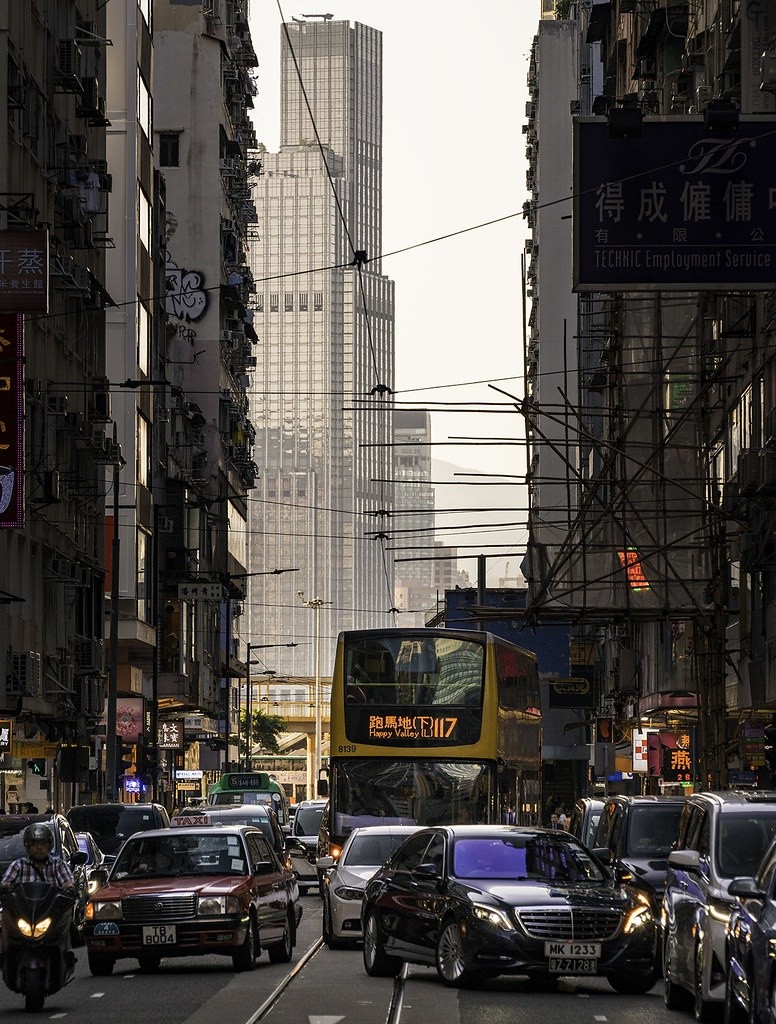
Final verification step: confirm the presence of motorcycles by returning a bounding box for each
[0,882,82,1011]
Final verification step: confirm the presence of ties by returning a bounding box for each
[554,824,556,830]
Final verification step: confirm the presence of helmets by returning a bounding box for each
[24,823,54,847]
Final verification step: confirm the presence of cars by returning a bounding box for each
[290,802,329,894]
[724,831,776,1024]
[570,798,604,848]
[360,827,661,998]
[0,813,88,940]
[73,832,117,896]
[593,792,689,908]
[169,804,293,871]
[84,815,304,977]
[316,820,433,947]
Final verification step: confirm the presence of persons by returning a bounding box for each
[546,814,564,831]
[132,850,183,873]
[0,824,79,964]
[20,802,39,814]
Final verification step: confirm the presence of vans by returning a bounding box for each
[66,802,170,868]
[659,789,776,1019]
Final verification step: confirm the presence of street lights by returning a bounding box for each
[237,671,276,770]
[152,503,213,800]
[93,439,123,799]
[245,643,299,772]
[225,567,300,772]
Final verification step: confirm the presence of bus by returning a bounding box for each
[239,756,329,811]
[206,773,290,840]
[318,627,542,864]
